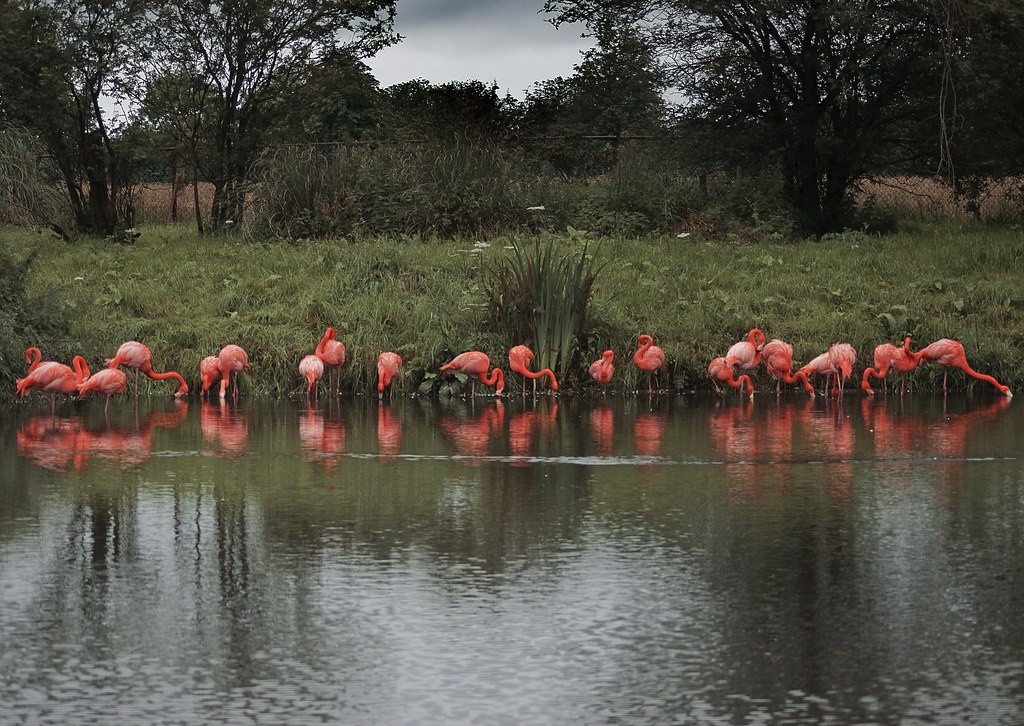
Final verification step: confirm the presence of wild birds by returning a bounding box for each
[440,351,505,400]
[633,335,666,391]
[15,341,188,412]
[861,337,1013,397]
[588,349,615,396]
[509,345,558,396]
[299,328,346,402]
[377,352,402,400]
[199,344,250,399]
[706,329,856,398]
